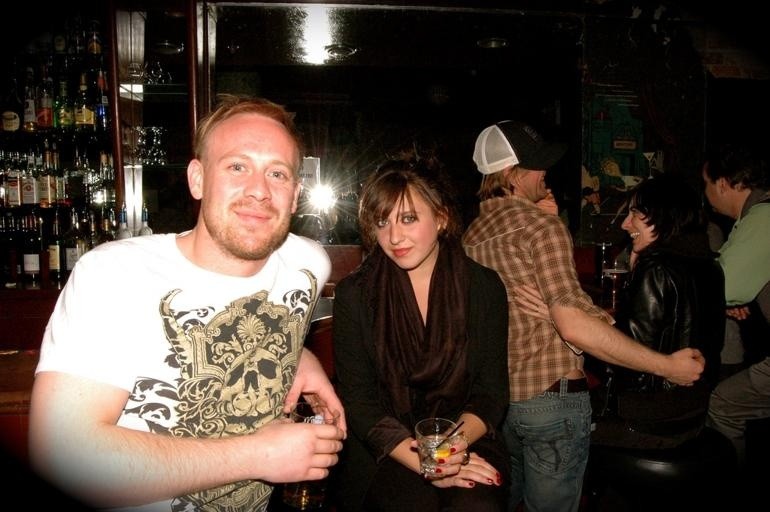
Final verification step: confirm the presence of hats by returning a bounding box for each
[473,119,565,174]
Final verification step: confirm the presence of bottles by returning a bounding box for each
[594,231,613,280]
[0,13,154,288]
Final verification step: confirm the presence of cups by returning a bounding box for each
[413,415,460,479]
[601,268,628,289]
[283,401,342,512]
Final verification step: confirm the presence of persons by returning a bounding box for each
[582,185,601,215]
[591,178,716,512]
[461,117,707,512]
[330,157,511,510]
[27,95,348,512]
[701,143,770,468]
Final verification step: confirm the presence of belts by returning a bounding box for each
[547,377,590,393]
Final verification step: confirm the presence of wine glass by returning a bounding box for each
[127,125,170,167]
